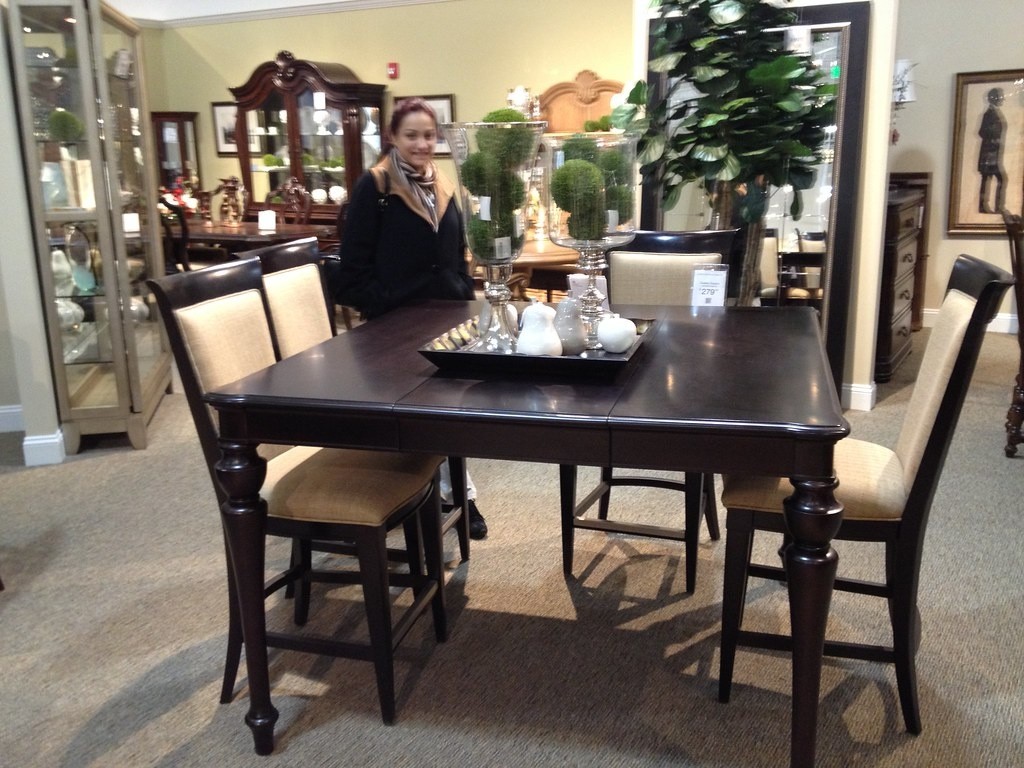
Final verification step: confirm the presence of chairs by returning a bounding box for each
[154,175,474,724]
[560,228,750,592]
[759,228,829,309]
[717,254,1016,735]
[998,205,1024,456]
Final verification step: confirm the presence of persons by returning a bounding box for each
[333,97,489,540]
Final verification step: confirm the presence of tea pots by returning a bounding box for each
[553,290,588,356]
[515,302,563,355]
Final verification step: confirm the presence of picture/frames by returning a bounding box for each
[211,101,264,157]
[393,93,457,158]
[947,68,1024,236]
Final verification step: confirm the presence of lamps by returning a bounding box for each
[892,58,916,110]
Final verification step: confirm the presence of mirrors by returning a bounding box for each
[648,1,871,400]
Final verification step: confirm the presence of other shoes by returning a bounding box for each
[451,498,490,540]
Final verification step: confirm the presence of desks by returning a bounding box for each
[162,217,337,248]
[202,297,852,768]
[463,235,578,300]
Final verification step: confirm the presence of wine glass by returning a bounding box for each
[543,133,643,359]
[439,121,548,356]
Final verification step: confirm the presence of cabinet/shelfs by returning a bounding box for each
[875,172,933,384]
[148,110,200,191]
[5,0,172,450]
[228,49,386,222]
